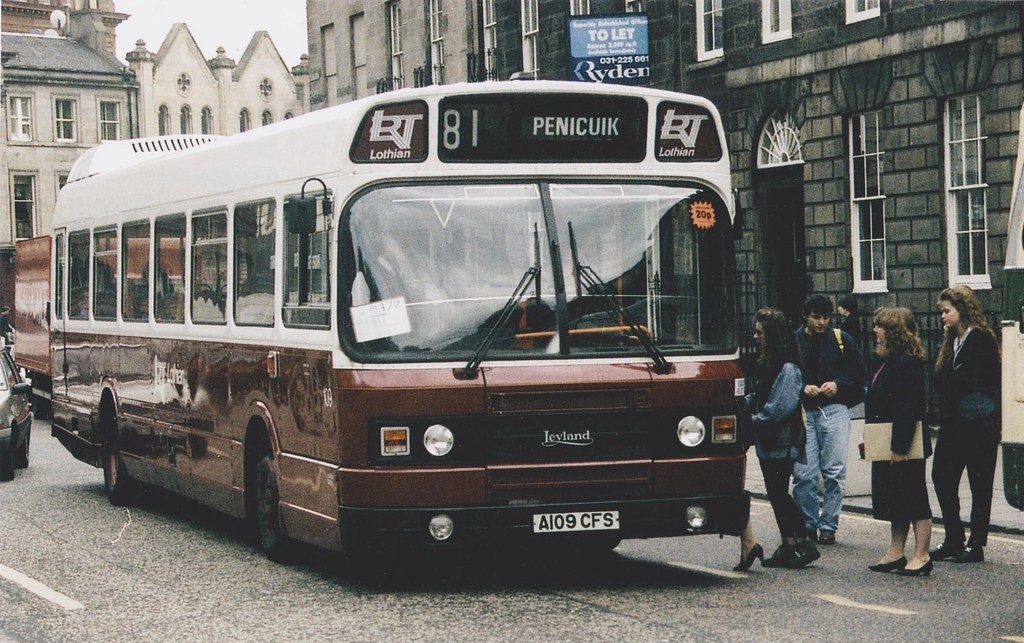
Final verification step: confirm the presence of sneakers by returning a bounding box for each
[951,542,983,561]
[929,538,964,560]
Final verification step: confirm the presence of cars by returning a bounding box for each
[0,345,36,482]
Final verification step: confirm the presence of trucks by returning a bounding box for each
[0,232,200,421]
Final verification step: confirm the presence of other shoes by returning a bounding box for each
[784,539,820,569]
[806,529,818,542]
[818,530,835,544]
[761,543,798,567]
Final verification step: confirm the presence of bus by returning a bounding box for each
[47,75,753,564]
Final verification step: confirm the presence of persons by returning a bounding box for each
[729,285,1002,576]
[350,226,429,346]
[71,257,281,326]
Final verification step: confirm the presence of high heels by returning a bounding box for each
[895,561,933,577]
[868,556,907,572]
[733,543,763,571]
